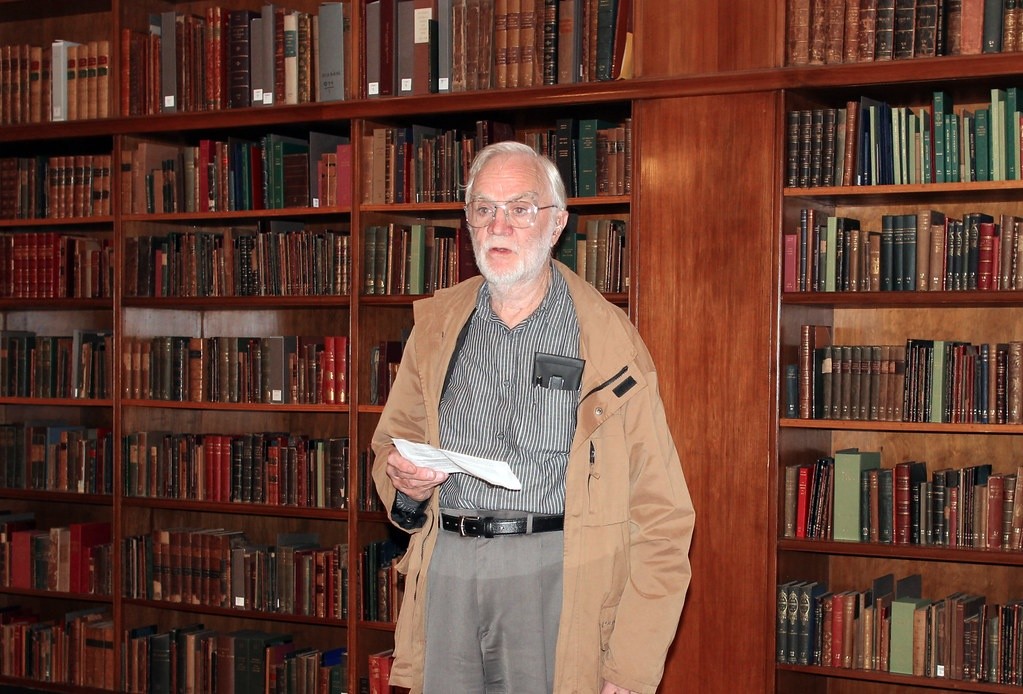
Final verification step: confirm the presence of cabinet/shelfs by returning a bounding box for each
[0,0,1023,694]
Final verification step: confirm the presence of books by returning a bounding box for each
[777,574,1023,690]
[359,539,408,622]
[0,608,351,694]
[784,449,1023,553]
[122,0,642,116]
[1,328,351,404]
[0,132,358,218]
[1,421,386,515]
[785,0,1023,67]
[360,648,393,693]
[364,119,631,300]
[0,231,353,300]
[785,325,1022,426]
[0,41,109,126]
[369,337,408,407]
[785,207,1023,294]
[788,85,1023,188]
[0,516,350,621]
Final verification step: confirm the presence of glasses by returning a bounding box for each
[463,199,559,228]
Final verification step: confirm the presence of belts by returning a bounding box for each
[439,511,564,538]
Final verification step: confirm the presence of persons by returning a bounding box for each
[370,140,694,694]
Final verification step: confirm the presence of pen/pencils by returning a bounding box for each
[536,376,543,387]
